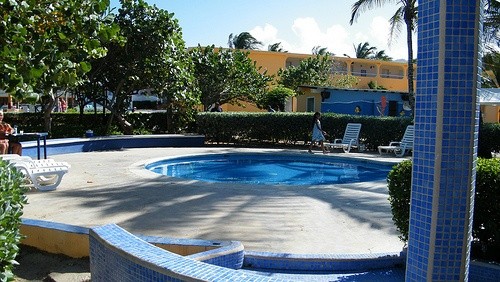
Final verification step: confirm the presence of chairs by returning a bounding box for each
[327,123,361,153]
[0,154,71,191]
[378,125,414,157]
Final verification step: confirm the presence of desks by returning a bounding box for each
[13,133,48,160]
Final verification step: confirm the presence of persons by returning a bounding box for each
[211,102,223,112]
[56,97,68,112]
[0,110,9,154]
[3,121,22,156]
[307,111,330,154]
[267,105,276,112]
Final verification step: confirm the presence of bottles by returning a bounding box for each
[14,125,17,134]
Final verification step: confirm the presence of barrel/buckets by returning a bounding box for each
[86,130,93,138]
